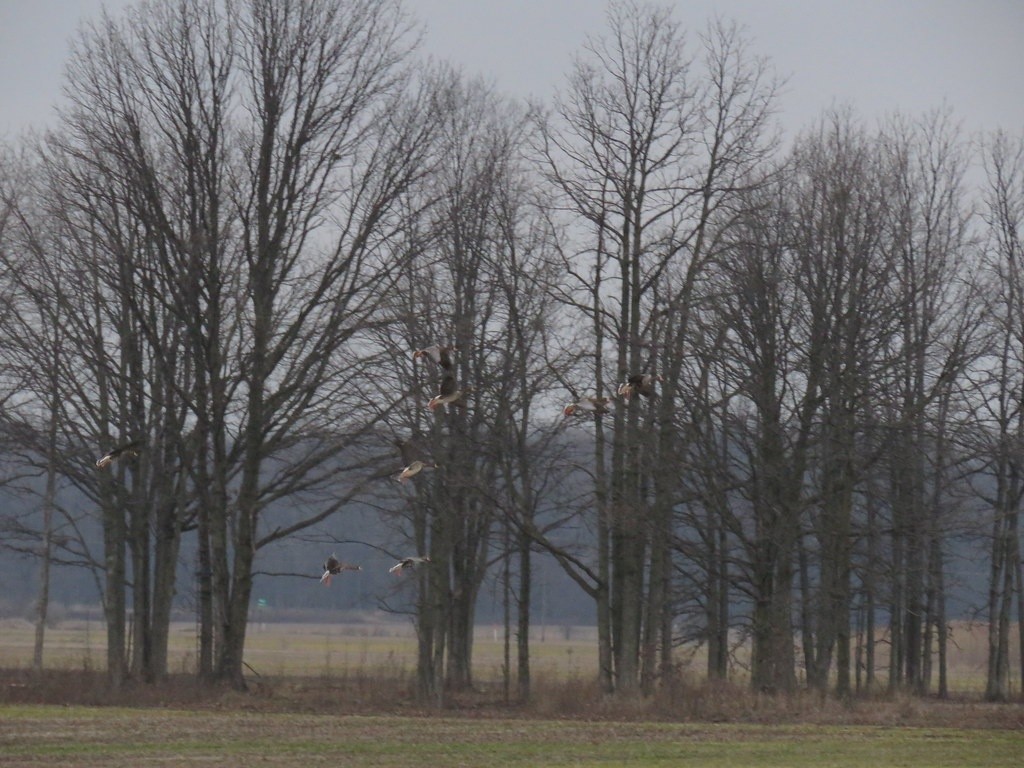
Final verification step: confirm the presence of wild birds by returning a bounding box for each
[96,438,142,469]
[616,374,662,398]
[413,345,464,408]
[318,557,362,585]
[397,442,437,480]
[565,396,611,417]
[388,554,432,573]
